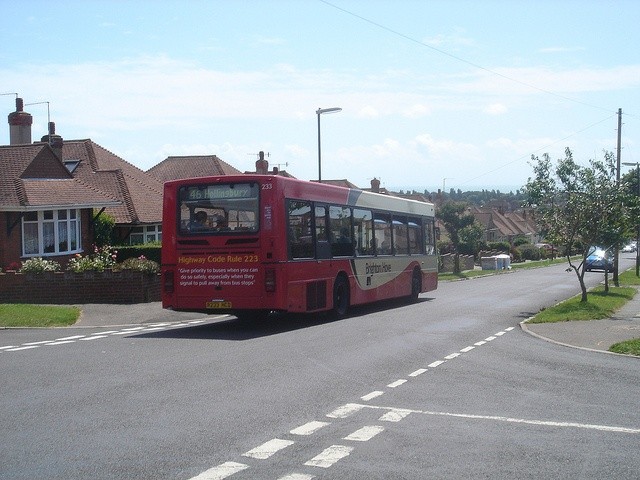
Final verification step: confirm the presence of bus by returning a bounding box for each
[161,174,440,317]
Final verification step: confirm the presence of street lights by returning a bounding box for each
[622,162,640,276]
[316,107,342,183]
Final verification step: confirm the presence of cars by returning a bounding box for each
[623,246,634,252]
[583,246,615,272]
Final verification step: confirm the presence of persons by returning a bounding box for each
[362,237,382,255]
[341,227,356,248]
[212,215,231,231]
[184,210,210,230]
[380,239,394,255]
[321,224,336,255]
